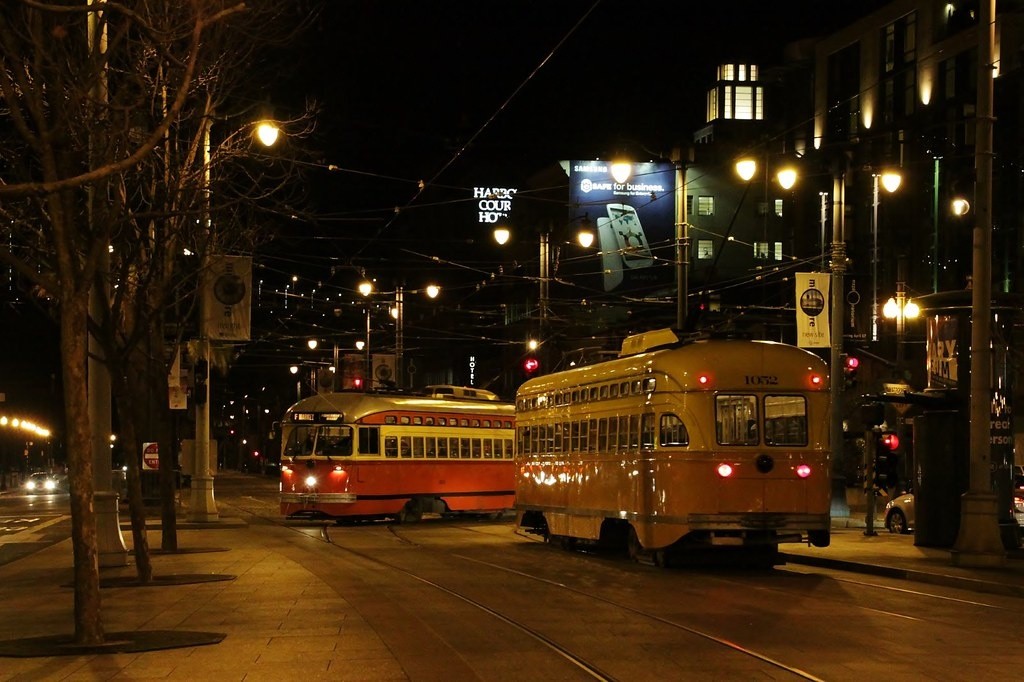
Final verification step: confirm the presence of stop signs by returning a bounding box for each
[142,443,159,469]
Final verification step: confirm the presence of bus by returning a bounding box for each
[512,326,832,565]
[269,386,516,525]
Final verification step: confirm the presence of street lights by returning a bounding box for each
[883,295,922,384]
[308,339,364,391]
[607,139,687,327]
[187,118,282,522]
[735,158,798,239]
[289,364,336,396]
[359,282,439,386]
[872,170,902,341]
[493,213,594,320]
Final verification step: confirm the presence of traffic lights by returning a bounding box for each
[524,359,538,374]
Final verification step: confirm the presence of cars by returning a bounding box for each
[26,472,53,495]
[883,487,915,534]
